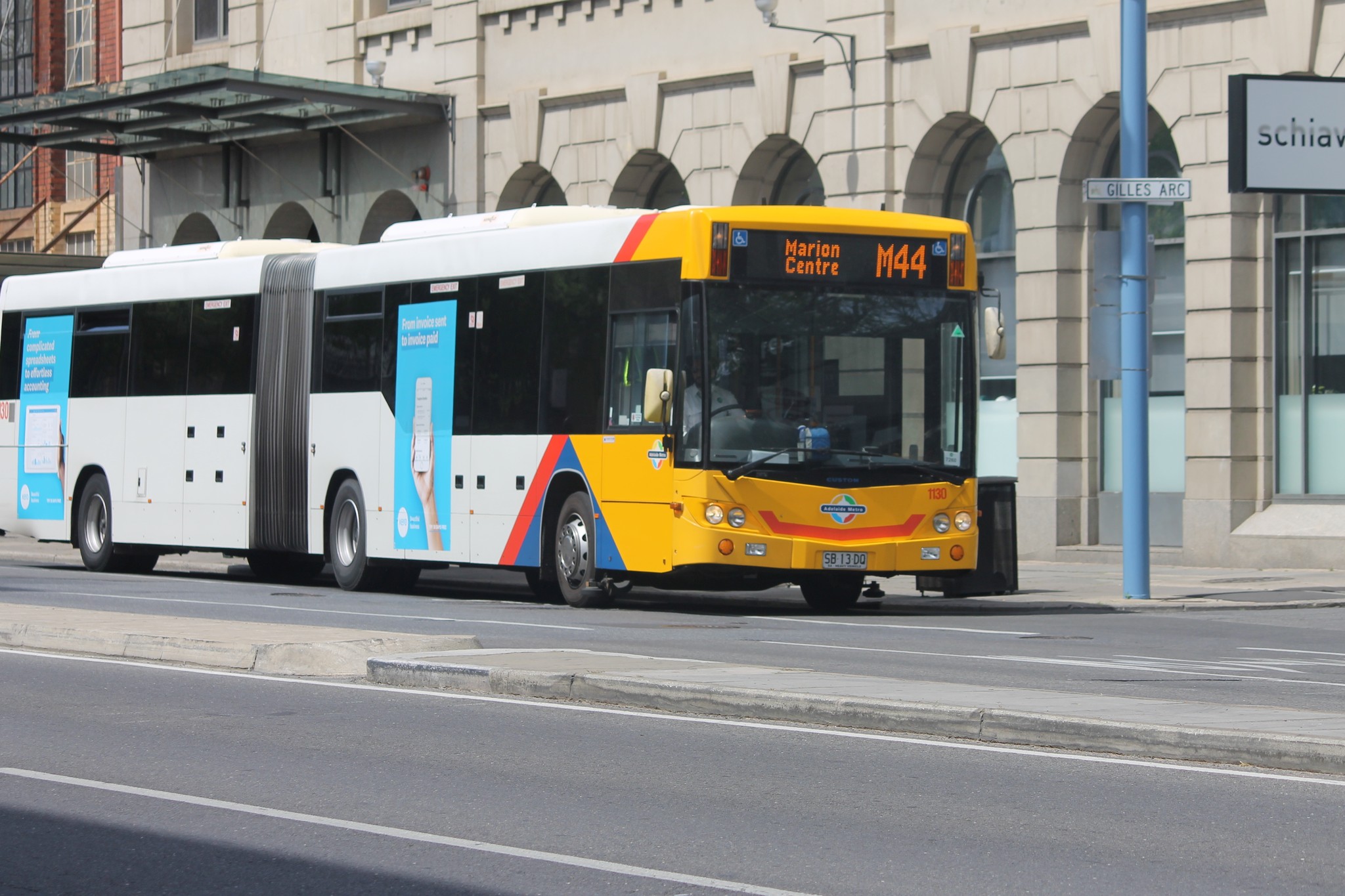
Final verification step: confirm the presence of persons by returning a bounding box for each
[670,357,750,446]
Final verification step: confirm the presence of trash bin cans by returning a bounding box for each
[916,474,1021,597]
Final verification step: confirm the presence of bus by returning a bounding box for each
[1,201,1006,611]
